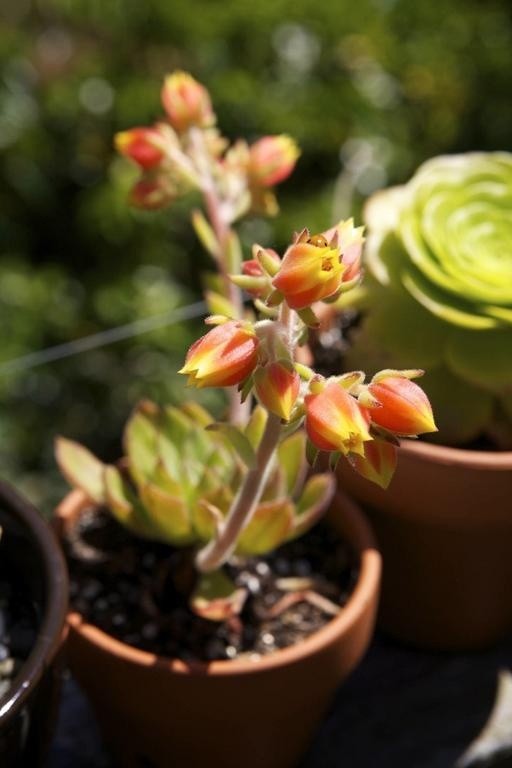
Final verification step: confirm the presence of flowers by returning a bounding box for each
[54,65,440,618]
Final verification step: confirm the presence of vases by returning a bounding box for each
[0,442,381,766]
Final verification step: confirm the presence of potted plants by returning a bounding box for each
[306,147,510,648]
